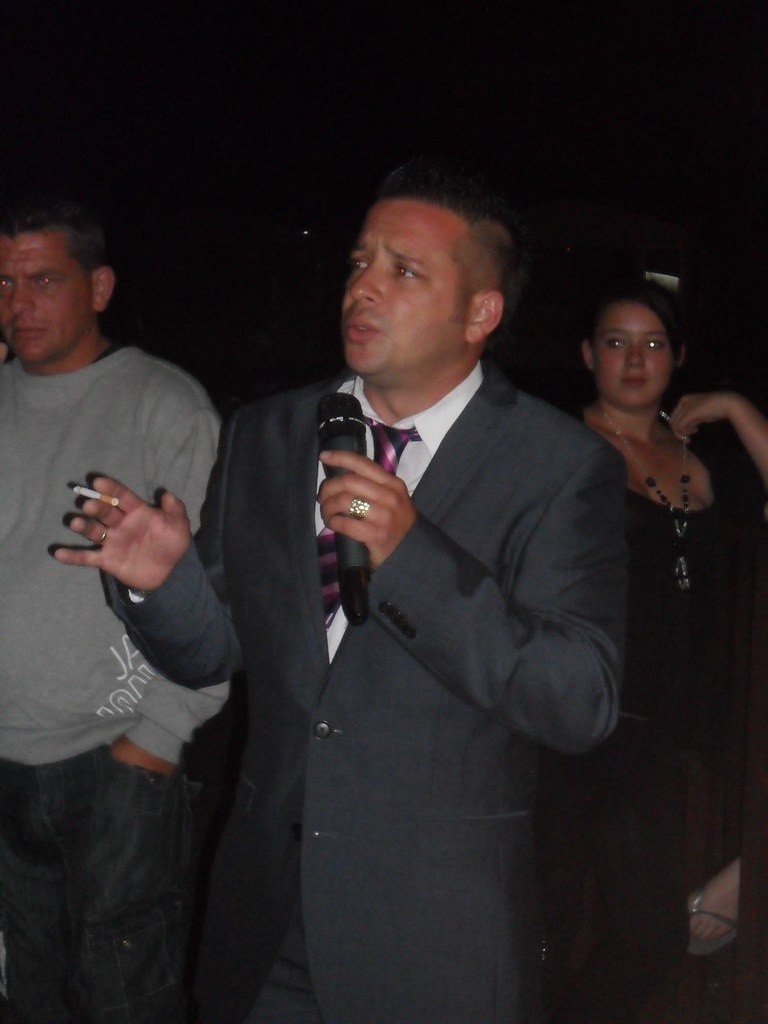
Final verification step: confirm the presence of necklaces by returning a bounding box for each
[599,400,693,591]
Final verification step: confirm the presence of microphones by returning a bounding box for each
[317,392,371,626]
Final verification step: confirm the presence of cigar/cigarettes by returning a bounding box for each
[73,485,119,507]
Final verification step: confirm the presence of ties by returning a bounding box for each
[316,417,423,629]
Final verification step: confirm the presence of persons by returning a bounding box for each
[53,170,628,1022]
[685,854,741,957]
[0,195,231,1024]
[533,279,768,1023]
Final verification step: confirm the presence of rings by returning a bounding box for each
[350,496,370,519]
[100,529,107,544]
